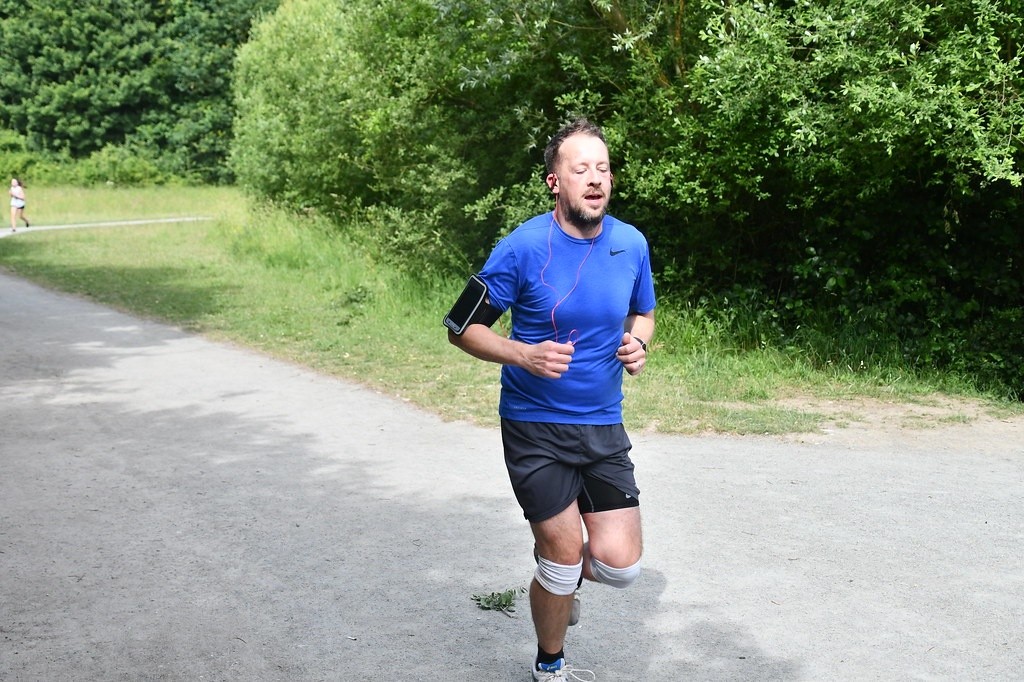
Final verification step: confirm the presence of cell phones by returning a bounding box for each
[443,273,487,336]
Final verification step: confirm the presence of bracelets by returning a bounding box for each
[634,337,647,353]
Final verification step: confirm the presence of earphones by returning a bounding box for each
[551,177,558,187]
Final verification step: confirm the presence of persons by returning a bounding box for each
[447,120,657,682]
[8,177,29,233]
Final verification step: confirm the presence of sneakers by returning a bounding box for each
[532,651,596,682]
[534,543,583,625]
